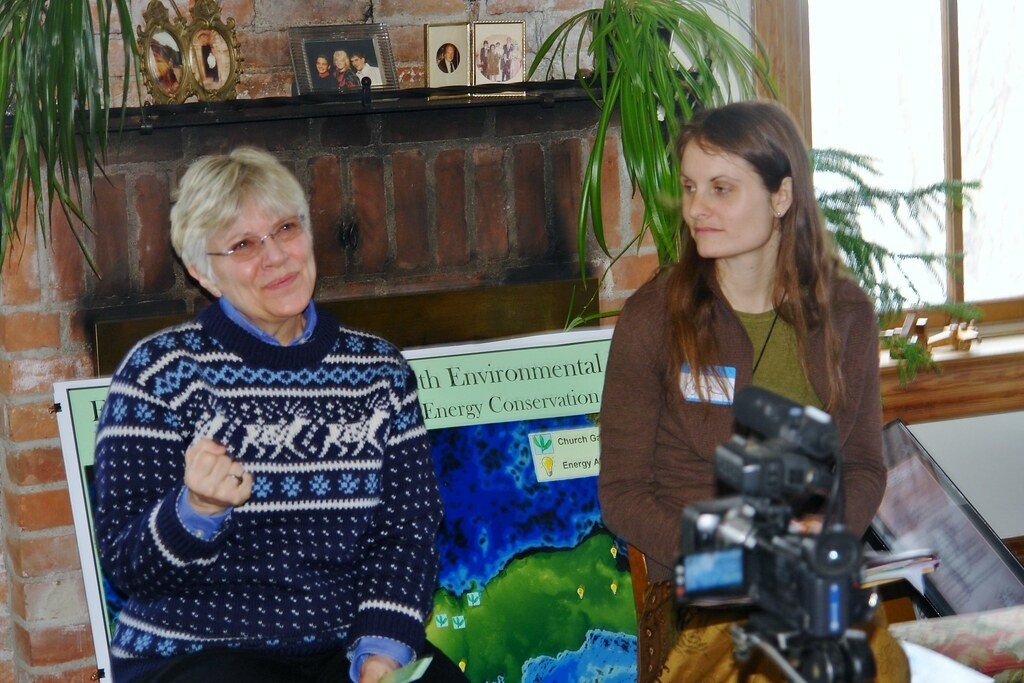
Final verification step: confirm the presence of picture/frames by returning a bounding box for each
[136,0,244,104]
[287,23,400,96]
[424,22,471,101]
[473,20,526,97]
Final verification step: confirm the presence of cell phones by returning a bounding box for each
[380,653,435,683]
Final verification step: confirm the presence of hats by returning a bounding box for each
[150,37,179,63]
[195,29,213,41]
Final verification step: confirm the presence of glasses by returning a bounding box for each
[205,215,304,264]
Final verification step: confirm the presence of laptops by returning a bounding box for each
[865,418,1024,620]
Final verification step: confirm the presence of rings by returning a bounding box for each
[226,473,242,486]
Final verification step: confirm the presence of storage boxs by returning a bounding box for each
[887,607,1024,683]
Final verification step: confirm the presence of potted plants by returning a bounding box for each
[531,0,980,377]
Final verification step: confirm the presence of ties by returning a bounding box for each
[450,62,454,73]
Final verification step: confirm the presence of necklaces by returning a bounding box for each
[751,288,787,376]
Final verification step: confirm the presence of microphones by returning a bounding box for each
[733,386,839,458]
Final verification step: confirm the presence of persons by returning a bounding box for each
[599,102,993,683]
[94,146,474,683]
[197,31,220,82]
[314,50,383,94]
[150,41,179,84]
[438,44,456,72]
[480,36,515,82]
